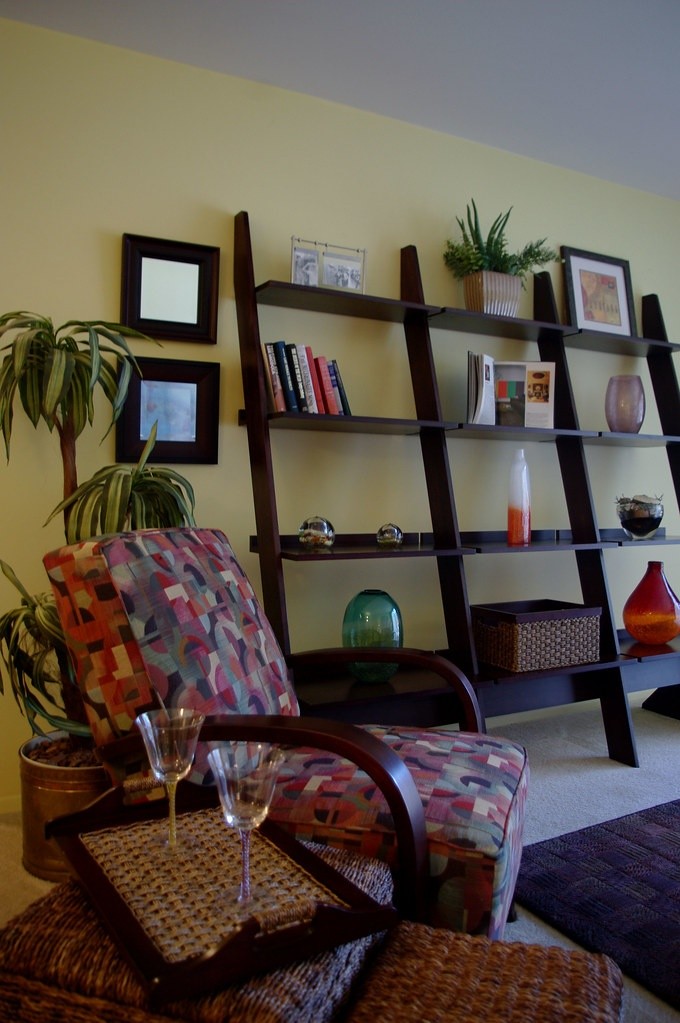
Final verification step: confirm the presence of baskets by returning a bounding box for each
[472,598,602,672]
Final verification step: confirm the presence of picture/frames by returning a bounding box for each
[116,233,218,345]
[115,355,221,465]
[560,245,637,338]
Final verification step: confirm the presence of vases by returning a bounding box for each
[605,375,645,434]
[622,561,680,646]
[508,450,532,546]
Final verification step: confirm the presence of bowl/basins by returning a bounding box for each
[616,503,664,540]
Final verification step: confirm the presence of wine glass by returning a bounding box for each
[206,744,286,919]
[135,708,206,854]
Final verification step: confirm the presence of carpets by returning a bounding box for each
[514,799,680,1014]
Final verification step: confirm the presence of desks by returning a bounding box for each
[0,885,623,1023]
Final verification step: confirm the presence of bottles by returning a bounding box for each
[605,375,646,434]
[342,589,403,649]
[623,561,680,647]
[506,448,532,547]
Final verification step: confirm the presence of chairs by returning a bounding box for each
[43,529,530,945]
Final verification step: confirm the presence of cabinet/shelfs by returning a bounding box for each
[235,209,680,769]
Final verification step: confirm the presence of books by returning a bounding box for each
[259,340,351,416]
[466,350,556,430]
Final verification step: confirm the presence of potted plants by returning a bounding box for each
[442,199,566,318]
[0,310,198,885]
[614,493,665,541]
